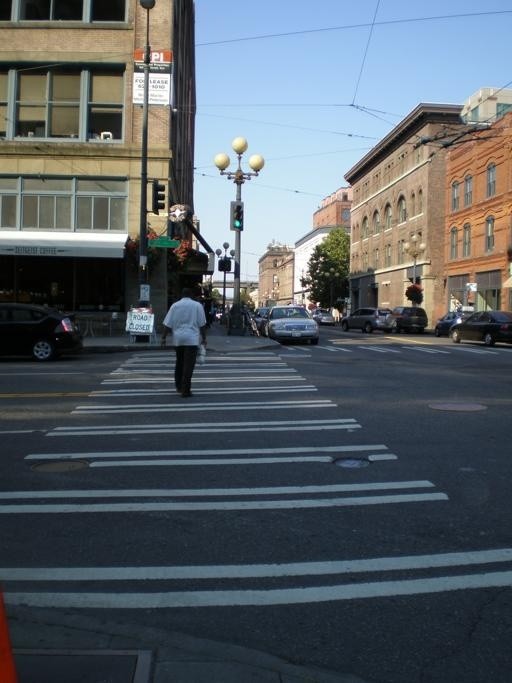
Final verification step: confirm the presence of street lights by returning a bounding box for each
[214,137,280,314]
[404,235,427,306]
[136,0,156,342]
[262,288,280,309]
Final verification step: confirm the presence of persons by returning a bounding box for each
[160,286,208,397]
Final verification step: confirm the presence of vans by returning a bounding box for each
[342,307,428,333]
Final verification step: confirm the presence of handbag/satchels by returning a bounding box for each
[196,345,207,365]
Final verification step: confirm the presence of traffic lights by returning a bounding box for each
[152,178,165,215]
[230,201,244,231]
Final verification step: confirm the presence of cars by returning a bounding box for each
[255,306,320,345]
[312,309,335,326]
[435,310,512,346]
[0,301,84,361]
